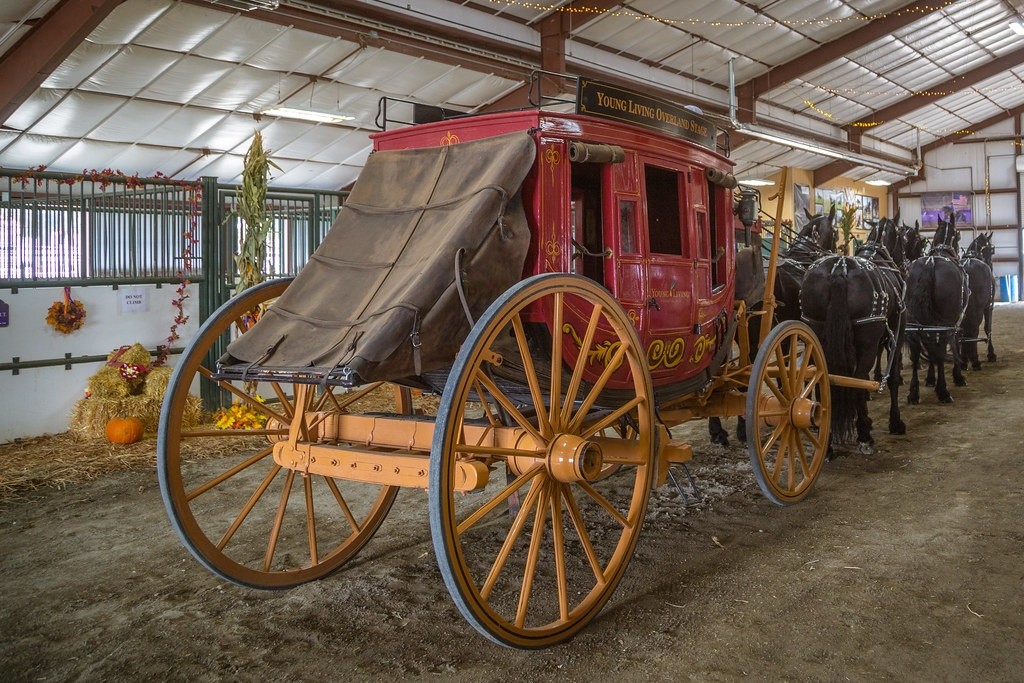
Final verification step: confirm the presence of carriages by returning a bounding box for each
[159,71,998,649]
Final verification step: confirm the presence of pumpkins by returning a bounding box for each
[107,411,144,443]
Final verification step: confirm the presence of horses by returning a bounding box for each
[708,205,997,462]
